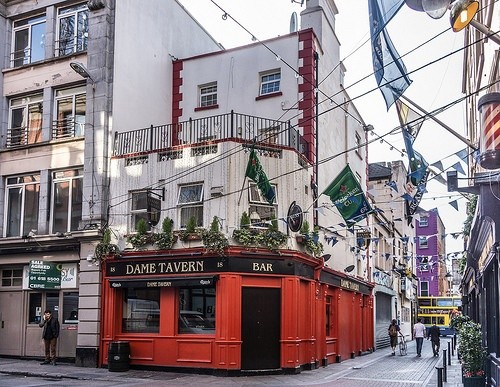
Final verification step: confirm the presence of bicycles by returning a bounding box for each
[398,334,410,356]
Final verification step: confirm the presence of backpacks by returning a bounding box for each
[390,326,397,336]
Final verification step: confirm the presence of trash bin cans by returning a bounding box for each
[108,341,131,372]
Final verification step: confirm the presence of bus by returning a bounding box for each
[417,296,462,308]
[418,306,459,337]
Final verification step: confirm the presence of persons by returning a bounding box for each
[413,318,426,357]
[388,319,404,355]
[427,321,441,358]
[39,310,60,365]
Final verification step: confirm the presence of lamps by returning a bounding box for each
[405,0,479,34]
[321,254,332,261]
[69,62,96,90]
[363,124,374,131]
[447,170,480,194]
[344,265,355,272]
[477,92,500,169]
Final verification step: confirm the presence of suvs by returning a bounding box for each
[126,308,217,336]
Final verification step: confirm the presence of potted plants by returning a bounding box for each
[456,314,488,387]
[96,215,325,257]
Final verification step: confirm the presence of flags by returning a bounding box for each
[322,164,375,228]
[403,153,431,226]
[367,0,414,112]
[245,151,276,206]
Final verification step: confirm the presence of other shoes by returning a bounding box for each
[51,361,57,366]
[392,351,395,356]
[434,351,439,356]
[40,362,48,365]
